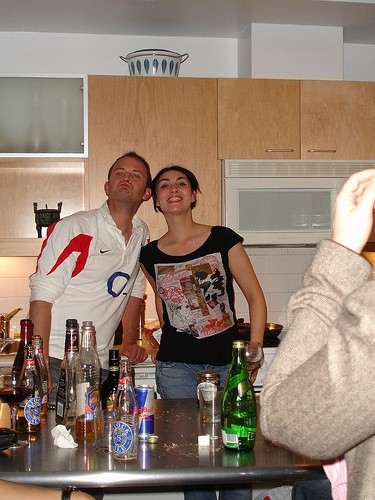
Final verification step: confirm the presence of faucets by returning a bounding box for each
[0,306,21,341]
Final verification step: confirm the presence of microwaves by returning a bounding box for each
[217,160,375,245]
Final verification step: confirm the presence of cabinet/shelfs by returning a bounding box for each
[217,78,375,160]
[0,73,88,256]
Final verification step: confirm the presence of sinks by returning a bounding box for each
[0,340,21,355]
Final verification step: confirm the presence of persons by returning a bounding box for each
[259,168,375,500]
[138,164,267,500]
[28,151,152,404]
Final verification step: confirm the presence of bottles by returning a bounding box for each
[31,335,49,417]
[111,359,140,464]
[100,349,121,413]
[55,319,80,428]
[221,340,257,451]
[74,321,104,441]
[10,319,40,444]
[197,369,221,425]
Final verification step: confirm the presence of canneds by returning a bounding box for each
[137,436,154,470]
[130,385,154,437]
[196,371,221,407]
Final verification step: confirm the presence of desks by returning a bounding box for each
[0,398,329,500]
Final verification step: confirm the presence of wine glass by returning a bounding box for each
[0,365,35,450]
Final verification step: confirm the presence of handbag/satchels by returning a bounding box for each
[0,427,18,451]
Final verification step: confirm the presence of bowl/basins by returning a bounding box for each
[243,323,282,337]
[119,48,189,77]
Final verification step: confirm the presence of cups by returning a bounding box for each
[94,410,117,455]
